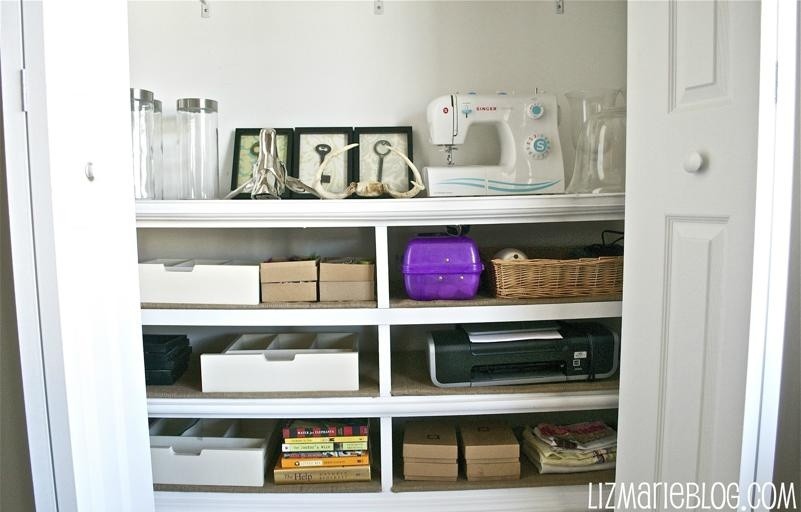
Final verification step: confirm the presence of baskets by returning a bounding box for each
[479,234,624,300]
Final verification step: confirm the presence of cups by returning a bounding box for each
[556,107,624,197]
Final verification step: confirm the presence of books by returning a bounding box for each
[270,418,376,488]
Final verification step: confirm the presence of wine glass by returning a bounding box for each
[562,88,622,196]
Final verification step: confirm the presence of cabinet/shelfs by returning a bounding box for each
[134,194,625,512]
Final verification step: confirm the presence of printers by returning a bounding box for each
[426,320,622,388]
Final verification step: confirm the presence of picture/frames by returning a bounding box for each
[231,127,414,199]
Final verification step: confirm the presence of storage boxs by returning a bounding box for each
[402,424,521,481]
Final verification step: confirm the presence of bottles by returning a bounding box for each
[127,86,220,201]
